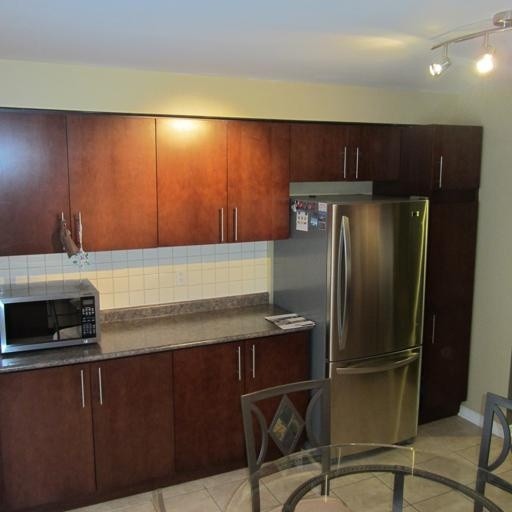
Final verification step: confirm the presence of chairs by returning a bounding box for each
[475,393,512,512]
[239,378,330,512]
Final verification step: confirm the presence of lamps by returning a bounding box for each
[428,25,512,77]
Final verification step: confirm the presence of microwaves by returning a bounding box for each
[1,278,99,358]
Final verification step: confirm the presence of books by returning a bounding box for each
[265,313,315,330]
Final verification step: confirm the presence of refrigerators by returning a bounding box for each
[271,193,432,459]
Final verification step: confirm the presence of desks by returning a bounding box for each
[222,441,512,512]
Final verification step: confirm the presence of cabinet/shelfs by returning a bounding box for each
[290,119,401,193]
[401,124,484,201]
[0,108,158,257]
[418,200,479,426]
[0,353,174,512]
[156,114,290,246]
[174,325,318,485]
